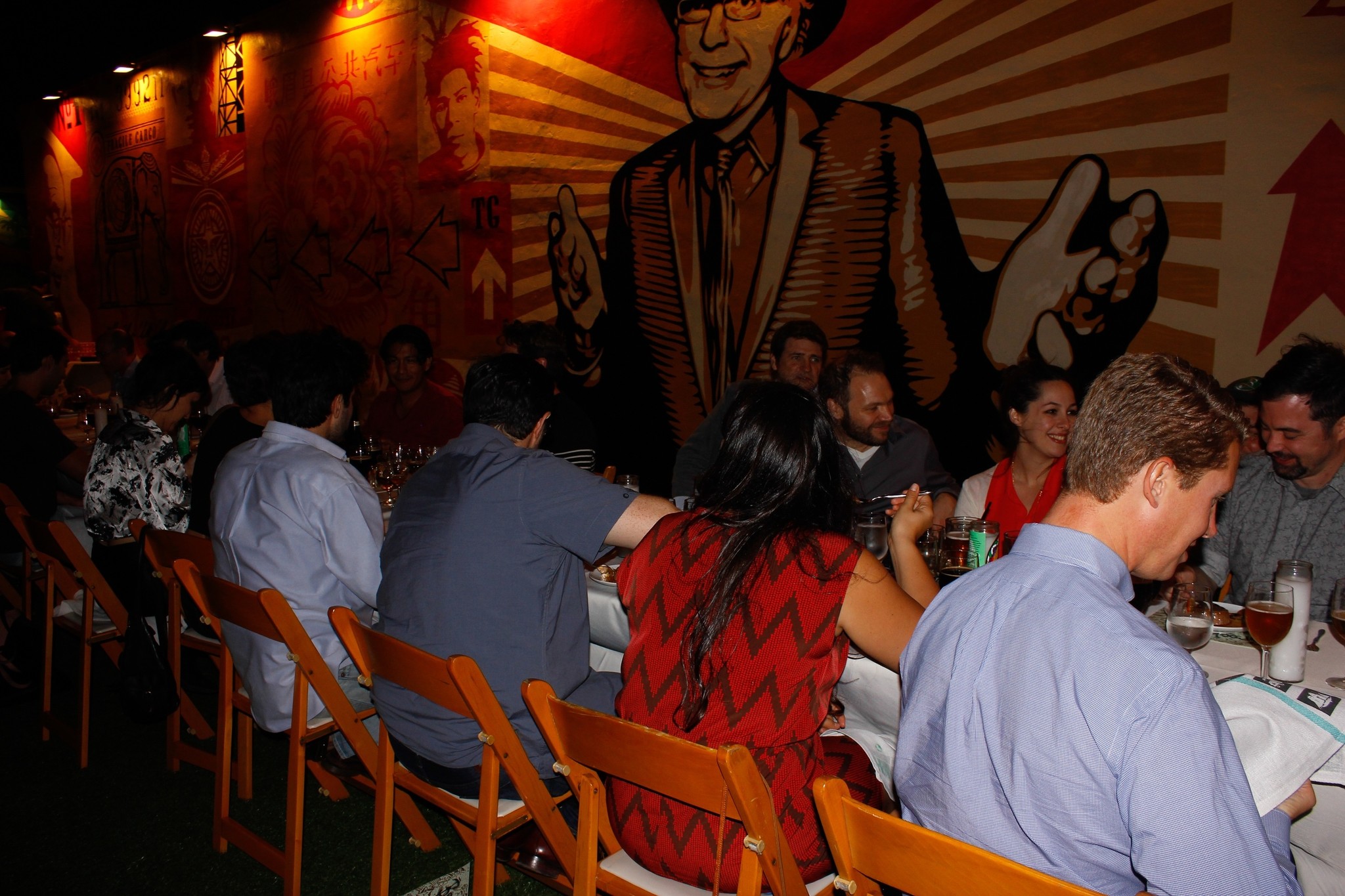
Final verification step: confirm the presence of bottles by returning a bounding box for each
[173,401,204,460]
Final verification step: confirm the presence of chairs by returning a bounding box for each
[814,771,1111,896]
[175,557,440,894]
[127,515,349,804]
[513,673,845,894]
[322,596,576,896]
[5,489,154,771]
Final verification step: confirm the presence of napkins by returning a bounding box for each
[1209,677,1344,824]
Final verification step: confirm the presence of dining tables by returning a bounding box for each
[580,508,1344,896]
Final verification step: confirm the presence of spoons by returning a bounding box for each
[1306,629,1327,652]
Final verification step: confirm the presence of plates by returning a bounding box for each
[590,561,624,586]
[1172,600,1249,633]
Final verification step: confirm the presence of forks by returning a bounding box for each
[850,489,931,504]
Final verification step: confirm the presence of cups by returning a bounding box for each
[851,511,890,562]
[917,514,1026,593]
[1268,559,1313,683]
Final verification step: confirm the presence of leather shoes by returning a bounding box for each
[518,826,605,880]
[494,829,526,863]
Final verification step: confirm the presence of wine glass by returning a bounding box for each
[1163,583,1215,680]
[77,400,95,448]
[1243,581,1294,683]
[340,424,442,511]
[1323,579,1345,692]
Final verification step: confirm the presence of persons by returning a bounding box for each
[890,348,1318,896]
[1,317,1345,689]
[208,324,397,786]
[366,352,681,878]
[605,372,940,896]
[0,235,50,333]
